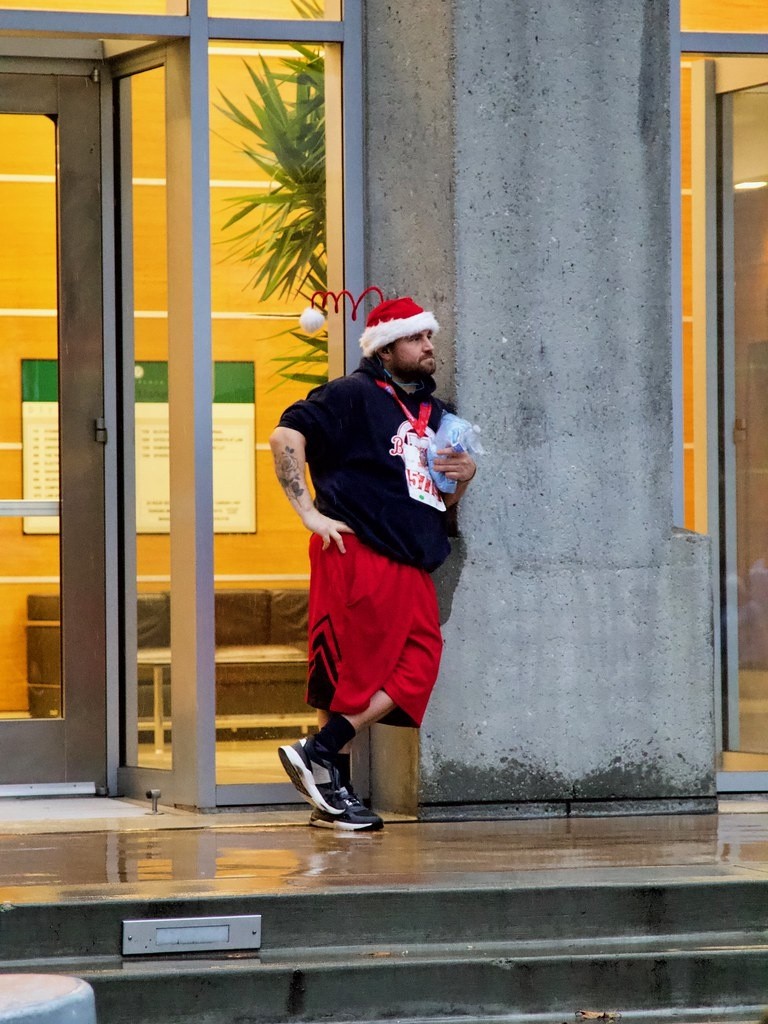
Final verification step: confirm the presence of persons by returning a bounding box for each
[268,296,478,831]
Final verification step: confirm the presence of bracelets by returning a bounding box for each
[454,459,476,483]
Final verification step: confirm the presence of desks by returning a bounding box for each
[136,646,319,754]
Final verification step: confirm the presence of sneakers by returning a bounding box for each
[278,734,349,814]
[309,785,384,831]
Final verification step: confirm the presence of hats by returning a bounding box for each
[301,284,440,358]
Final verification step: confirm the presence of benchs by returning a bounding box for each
[27,588,319,744]
